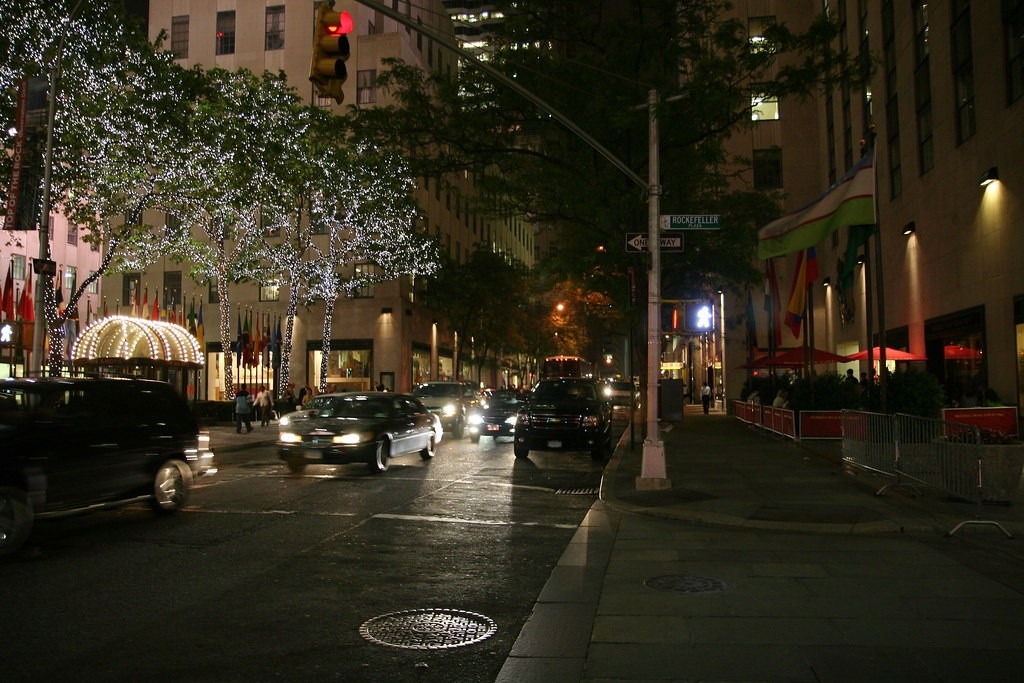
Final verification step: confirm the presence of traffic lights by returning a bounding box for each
[308,5,354,91]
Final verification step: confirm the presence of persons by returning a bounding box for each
[232,383,254,434]
[253,385,275,428]
[700,381,711,415]
[844,369,858,384]
[373,380,379,391]
[858,372,871,393]
[299,381,314,406]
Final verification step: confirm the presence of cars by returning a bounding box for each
[275,391,444,474]
[0,374,218,553]
[415,380,487,440]
[514,377,613,459]
[470,390,525,443]
[608,382,636,408]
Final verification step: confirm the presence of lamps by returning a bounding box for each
[822,276,830,287]
[976,167,999,186]
[901,220,916,236]
[856,254,865,265]
[382,307,392,314]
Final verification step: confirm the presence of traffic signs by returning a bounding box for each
[626,233,684,253]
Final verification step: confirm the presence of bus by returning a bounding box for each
[543,356,593,379]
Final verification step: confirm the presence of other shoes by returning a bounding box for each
[246,427,253,432]
[237,430,242,433]
[266,421,270,426]
[261,423,264,426]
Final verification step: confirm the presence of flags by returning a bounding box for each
[0,264,282,368]
[746,282,760,360]
[757,143,875,262]
[763,257,784,349]
[785,247,823,340]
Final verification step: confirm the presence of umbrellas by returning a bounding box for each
[839,345,929,362]
[945,345,985,359]
[733,346,852,384]
